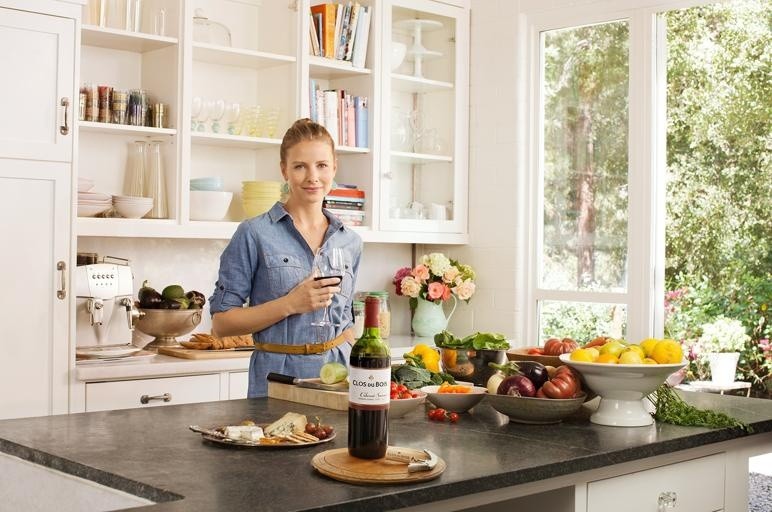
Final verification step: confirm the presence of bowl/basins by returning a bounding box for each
[440,347,507,386]
[114,193,153,219]
[422,384,489,414]
[191,179,222,189]
[389,389,428,419]
[240,178,283,216]
[505,346,560,365]
[391,41,406,74]
[484,389,590,424]
[189,190,233,222]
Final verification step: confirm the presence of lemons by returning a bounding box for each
[570,337,683,364]
[163,285,184,298]
[170,296,194,309]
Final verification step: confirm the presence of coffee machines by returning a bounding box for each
[77,253,140,359]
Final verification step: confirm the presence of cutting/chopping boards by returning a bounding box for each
[159,344,256,359]
[266,373,349,410]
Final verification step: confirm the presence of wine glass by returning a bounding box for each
[558,345,687,426]
[188,96,283,138]
[133,304,203,348]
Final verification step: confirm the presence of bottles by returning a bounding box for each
[353,290,395,339]
[78,84,168,129]
[150,140,169,218]
[132,140,148,197]
[348,296,392,459]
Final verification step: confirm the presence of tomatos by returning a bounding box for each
[544,338,578,355]
[536,365,582,399]
[426,408,458,423]
[390,382,418,400]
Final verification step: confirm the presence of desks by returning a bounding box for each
[694,377,753,398]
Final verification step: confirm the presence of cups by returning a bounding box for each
[396,202,451,220]
[705,352,740,382]
[385,100,443,154]
[87,0,109,28]
[148,5,169,36]
[119,0,140,30]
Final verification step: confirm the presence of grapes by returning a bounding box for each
[304,423,333,439]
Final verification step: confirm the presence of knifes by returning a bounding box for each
[204,345,255,352]
[266,371,348,393]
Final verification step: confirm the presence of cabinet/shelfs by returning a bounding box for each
[0,165,79,417]
[75,362,221,416]
[476,441,756,512]
[74,0,475,251]
[0,1,80,165]
[222,361,252,402]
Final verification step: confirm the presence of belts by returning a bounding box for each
[254,332,349,355]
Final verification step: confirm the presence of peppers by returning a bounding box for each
[403,344,440,374]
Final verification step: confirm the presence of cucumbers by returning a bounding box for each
[319,362,348,384]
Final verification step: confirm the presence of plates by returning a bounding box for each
[201,417,339,447]
[77,190,111,217]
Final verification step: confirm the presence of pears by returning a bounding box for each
[137,280,156,299]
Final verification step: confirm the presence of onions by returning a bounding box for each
[497,375,537,397]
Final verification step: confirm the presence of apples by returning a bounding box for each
[187,291,205,305]
[140,290,163,309]
[160,300,180,308]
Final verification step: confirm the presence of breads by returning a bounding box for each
[264,412,307,438]
[178,328,254,349]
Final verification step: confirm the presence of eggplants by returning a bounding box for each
[488,360,548,390]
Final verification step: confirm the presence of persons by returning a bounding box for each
[209,118,362,401]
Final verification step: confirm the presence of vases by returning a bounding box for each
[706,349,742,387]
[145,134,172,221]
[409,288,460,338]
[131,139,150,200]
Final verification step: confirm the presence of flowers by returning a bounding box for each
[390,249,477,310]
[694,313,750,355]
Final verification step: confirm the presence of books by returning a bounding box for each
[322,184,366,227]
[307,1,373,148]
